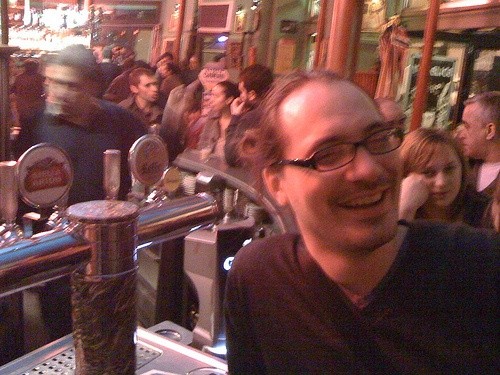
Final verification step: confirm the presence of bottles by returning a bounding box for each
[102,150,121,199]
[131,174,146,203]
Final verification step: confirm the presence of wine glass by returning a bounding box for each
[1,161,18,243]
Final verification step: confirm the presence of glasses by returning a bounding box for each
[277,126,403,172]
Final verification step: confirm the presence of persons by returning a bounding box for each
[9,44,500,342]
[224,71,500,375]
